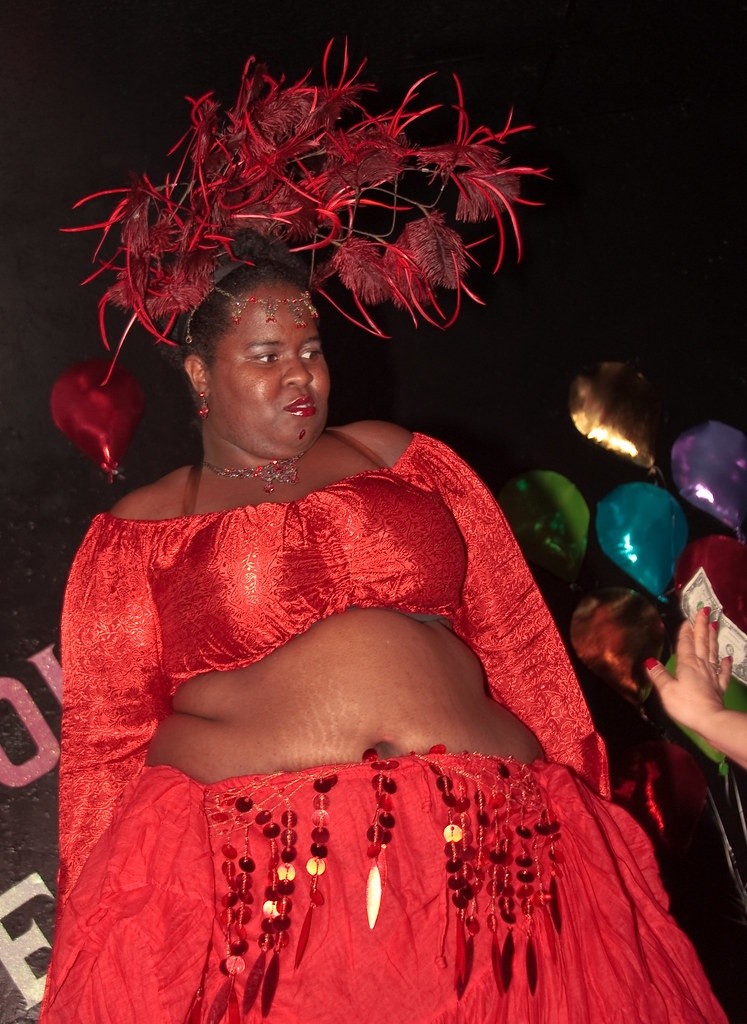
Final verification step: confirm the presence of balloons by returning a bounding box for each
[609,738,709,884]
[664,653,747,772]
[672,421,747,528]
[674,536,747,632]
[571,588,665,702]
[496,469,589,574]
[570,362,658,468]
[596,483,685,594]
[50,359,146,482]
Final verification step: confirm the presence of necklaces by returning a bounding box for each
[202,457,300,494]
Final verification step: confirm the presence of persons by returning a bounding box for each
[644,607,747,769]
[40,236,727,1024]
[570,590,747,1024]
